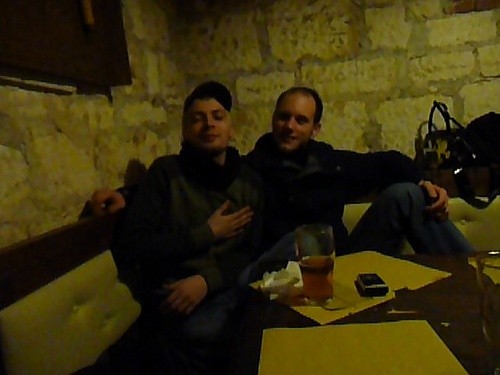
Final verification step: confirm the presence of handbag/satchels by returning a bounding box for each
[421,100,465,196]
[453,112,500,209]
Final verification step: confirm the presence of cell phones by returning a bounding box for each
[357,274,388,294]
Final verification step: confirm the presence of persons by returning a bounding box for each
[143,79,270,375]
[90,86,478,259]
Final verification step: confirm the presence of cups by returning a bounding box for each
[294,224,336,304]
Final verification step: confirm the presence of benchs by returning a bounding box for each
[1,168,500,375]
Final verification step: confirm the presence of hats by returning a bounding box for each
[184,81,232,111]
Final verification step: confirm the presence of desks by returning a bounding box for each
[245,255,500,375]
